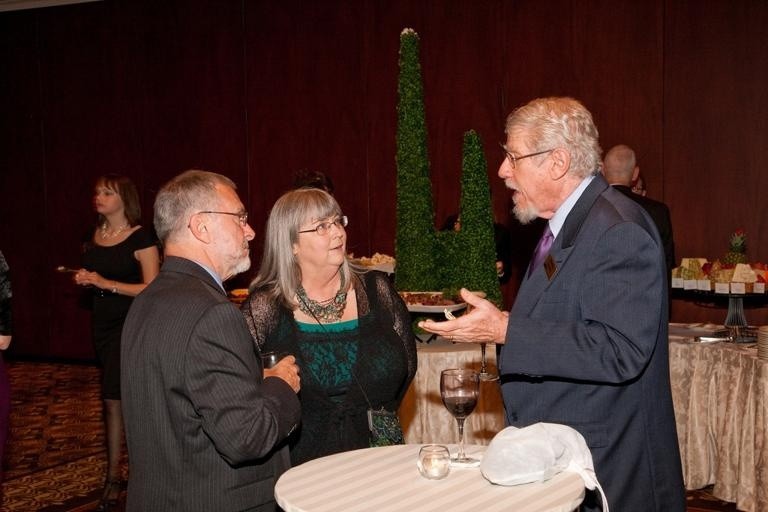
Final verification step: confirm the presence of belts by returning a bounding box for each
[441,370,482,465]
[464,302,500,383]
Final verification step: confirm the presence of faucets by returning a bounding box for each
[96,477,125,512]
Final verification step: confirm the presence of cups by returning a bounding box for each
[188,211,248,228]
[498,142,554,169]
[298,216,348,235]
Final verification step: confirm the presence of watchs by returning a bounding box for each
[112,280,117,294]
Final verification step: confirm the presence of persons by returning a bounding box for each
[602,147,676,321]
[447,213,511,285]
[0,250,14,455]
[632,177,647,197]
[119,170,302,512]
[73,172,160,512]
[416,96,686,512]
[240,186,417,469]
[291,170,334,198]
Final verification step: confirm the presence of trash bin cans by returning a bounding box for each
[112,280,117,294]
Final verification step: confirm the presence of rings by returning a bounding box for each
[451,336,453,341]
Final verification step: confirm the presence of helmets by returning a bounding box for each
[757,326,768,360]
[396,291,486,312]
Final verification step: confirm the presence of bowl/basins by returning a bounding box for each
[101,219,129,240]
[295,266,347,323]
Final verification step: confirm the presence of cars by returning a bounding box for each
[417,443,450,479]
[259,351,287,383]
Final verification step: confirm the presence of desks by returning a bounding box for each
[396,325,766,509]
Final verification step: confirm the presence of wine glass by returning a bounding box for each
[451,336,453,341]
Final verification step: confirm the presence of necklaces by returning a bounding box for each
[295,266,347,323]
[101,219,129,240]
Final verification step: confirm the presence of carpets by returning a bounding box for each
[528,223,553,277]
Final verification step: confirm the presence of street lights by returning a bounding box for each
[366,408,404,447]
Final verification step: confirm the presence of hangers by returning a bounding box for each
[55,266,81,274]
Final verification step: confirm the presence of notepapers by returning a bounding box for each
[723,228,748,265]
